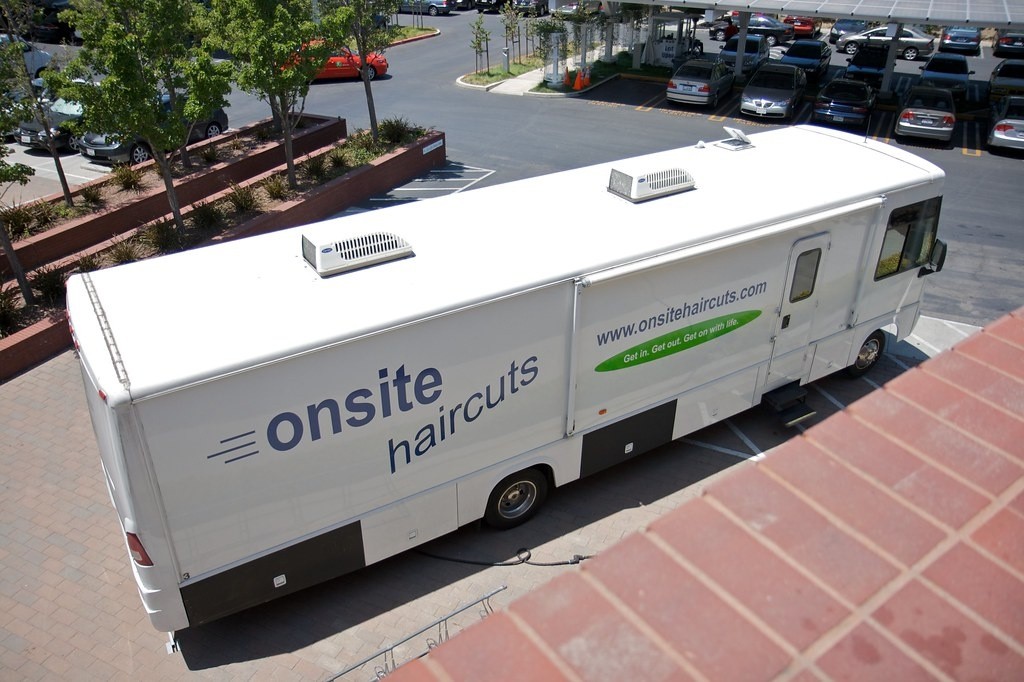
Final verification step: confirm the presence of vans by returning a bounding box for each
[0,31,59,81]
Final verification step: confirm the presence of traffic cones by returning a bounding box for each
[573,66,591,91]
[563,66,571,86]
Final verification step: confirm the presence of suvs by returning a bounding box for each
[845,43,898,90]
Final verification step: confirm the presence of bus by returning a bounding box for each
[65,122,947,655]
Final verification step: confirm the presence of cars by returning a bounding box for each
[448,0,549,17]
[666,58,737,110]
[983,94,1024,154]
[717,33,770,78]
[986,58,1024,106]
[556,1,623,24]
[77,93,229,165]
[991,28,1024,59]
[894,84,960,147]
[937,25,985,55]
[274,38,389,84]
[812,77,880,132]
[386,0,458,16]
[784,14,823,38]
[14,96,93,154]
[740,62,807,123]
[829,19,871,44]
[708,14,794,47]
[780,39,832,83]
[919,51,975,104]
[0,77,87,138]
[836,24,935,61]
